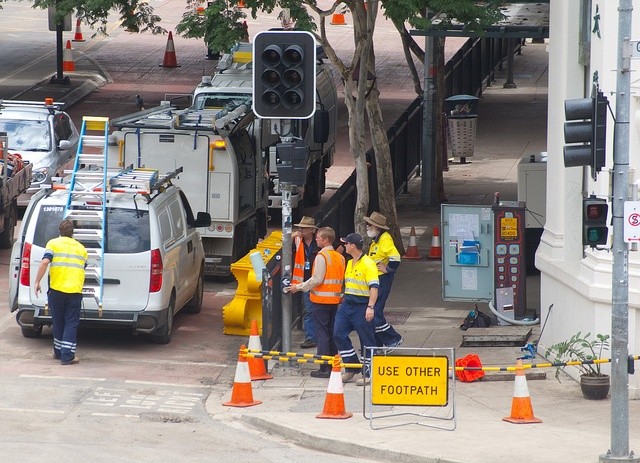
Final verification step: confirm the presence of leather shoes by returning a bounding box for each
[356,376,371,386]
[340,371,363,382]
[383,334,404,354]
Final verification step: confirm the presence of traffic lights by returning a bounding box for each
[564,83,608,181]
[254,31,316,119]
[583,196,608,246]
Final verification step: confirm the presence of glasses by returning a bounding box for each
[366,221,373,227]
[344,241,349,245]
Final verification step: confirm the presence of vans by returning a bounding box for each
[105,98,270,283]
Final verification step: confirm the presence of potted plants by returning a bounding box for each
[545,331,610,398]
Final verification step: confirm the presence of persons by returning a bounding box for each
[287,227,346,378]
[363,211,404,354]
[34,221,88,365]
[291,216,322,348]
[332,233,380,386]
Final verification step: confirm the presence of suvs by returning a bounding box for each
[8,118,211,344]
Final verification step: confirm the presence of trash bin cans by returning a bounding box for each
[445,95,480,164]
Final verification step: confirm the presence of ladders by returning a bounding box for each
[44,116,111,317]
[111,168,163,190]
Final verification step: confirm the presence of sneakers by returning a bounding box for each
[300,338,317,348]
[62,356,80,364]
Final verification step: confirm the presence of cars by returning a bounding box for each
[0,98,81,221]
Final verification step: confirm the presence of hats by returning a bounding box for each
[363,210,390,230]
[340,232,364,248]
[294,214,320,229]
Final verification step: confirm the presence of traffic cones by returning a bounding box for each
[63,40,74,71]
[316,356,353,419]
[247,320,273,381]
[401,227,423,259]
[502,360,543,424]
[72,19,86,41]
[236,0,245,9]
[159,31,181,67]
[221,345,262,407]
[330,4,347,24]
[197,0,206,18]
[427,225,441,259]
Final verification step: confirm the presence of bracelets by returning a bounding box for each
[367,306,374,309]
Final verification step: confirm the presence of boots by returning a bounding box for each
[310,363,332,378]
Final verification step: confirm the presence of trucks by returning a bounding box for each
[0,132,32,247]
[171,42,338,225]
